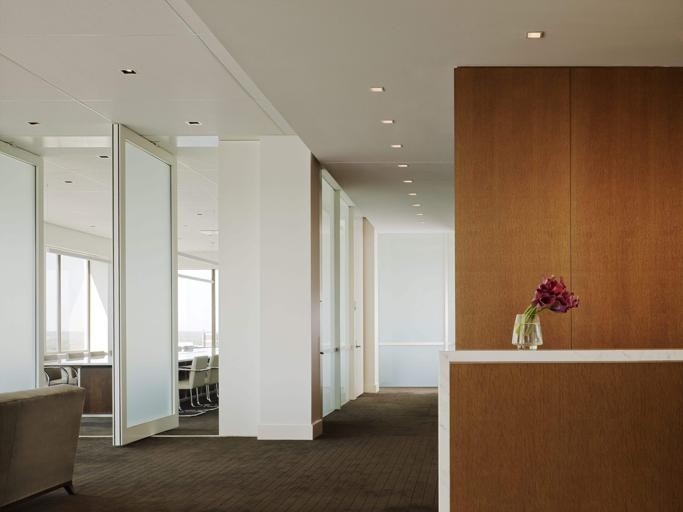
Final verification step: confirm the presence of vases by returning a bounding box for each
[511,312,543,350]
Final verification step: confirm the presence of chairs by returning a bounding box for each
[41,345,218,417]
[0,384,89,510]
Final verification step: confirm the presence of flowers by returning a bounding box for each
[514,273,580,345]
